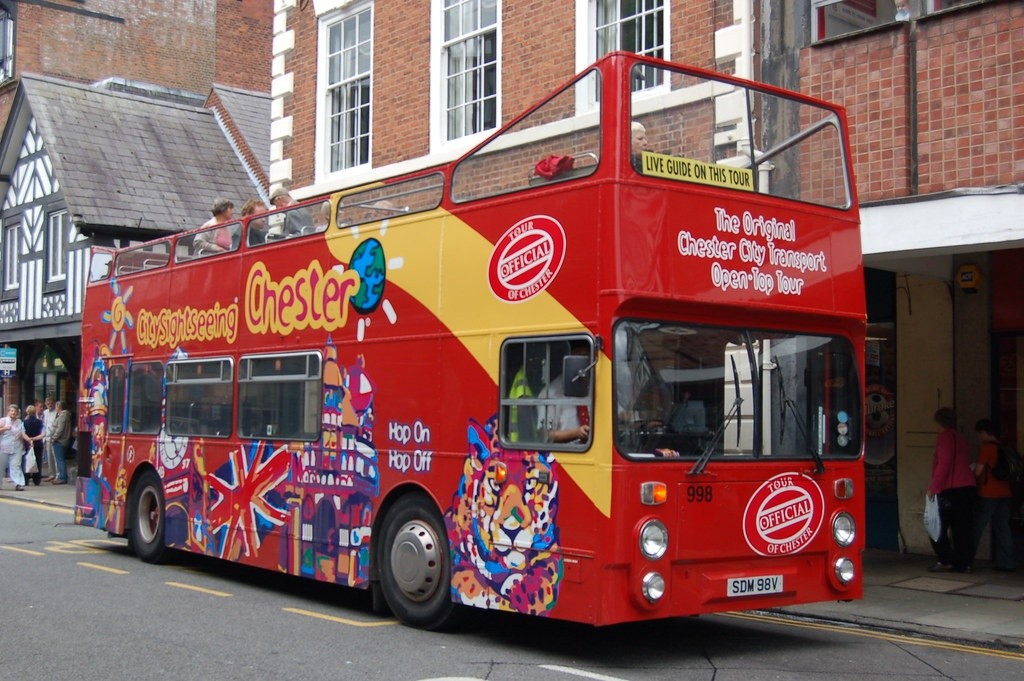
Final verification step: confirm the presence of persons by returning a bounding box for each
[925,406,979,574]
[631,121,654,175]
[972,417,1018,573]
[508,349,543,443]
[193,183,395,261]
[535,345,662,447]
[0,395,77,491]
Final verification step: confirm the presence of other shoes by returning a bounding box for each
[52,480,66,484]
[928,562,971,573]
[44,476,55,481]
[16,485,24,491]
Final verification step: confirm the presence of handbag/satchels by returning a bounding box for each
[25,447,38,473]
[925,490,942,543]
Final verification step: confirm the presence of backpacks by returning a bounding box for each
[984,440,1024,484]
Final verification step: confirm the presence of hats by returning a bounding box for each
[269,188,289,205]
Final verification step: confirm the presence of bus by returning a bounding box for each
[71,48,872,624]
